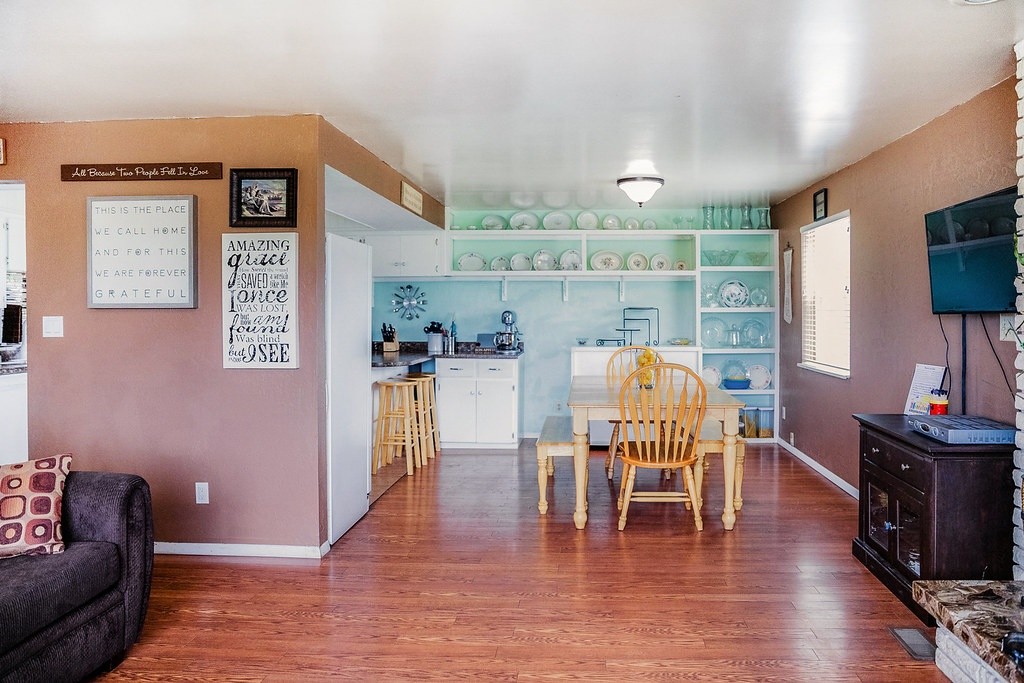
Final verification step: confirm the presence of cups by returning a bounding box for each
[427,333,443,356]
[930,400,949,416]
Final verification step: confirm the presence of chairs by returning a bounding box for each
[606,345,674,481]
[616,362,707,533]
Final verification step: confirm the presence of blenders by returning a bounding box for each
[492,310,523,355]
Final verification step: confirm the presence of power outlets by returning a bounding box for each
[781,406,785,420]
[790,432,794,447]
[195,482,209,504]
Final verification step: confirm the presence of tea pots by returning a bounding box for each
[450,223,533,230]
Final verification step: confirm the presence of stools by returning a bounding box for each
[375,371,440,476]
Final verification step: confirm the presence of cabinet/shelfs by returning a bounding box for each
[352,236,780,451]
[853,412,1019,628]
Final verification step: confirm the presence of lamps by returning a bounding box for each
[615,175,666,208]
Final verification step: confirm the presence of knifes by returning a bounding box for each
[381,323,394,342]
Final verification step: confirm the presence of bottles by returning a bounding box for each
[703,205,769,229]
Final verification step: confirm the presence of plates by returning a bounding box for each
[457,249,582,271]
[673,258,688,270]
[481,211,622,230]
[651,254,671,271]
[625,217,639,230]
[702,365,721,388]
[701,317,729,348]
[742,317,769,348]
[590,251,625,270]
[642,219,657,231]
[746,365,771,389]
[627,252,650,271]
[701,279,768,308]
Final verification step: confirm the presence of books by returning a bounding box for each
[912,580,1024,683]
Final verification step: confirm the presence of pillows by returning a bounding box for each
[1,452,73,560]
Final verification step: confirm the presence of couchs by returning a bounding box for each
[1,472,153,682]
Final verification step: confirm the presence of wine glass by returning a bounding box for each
[672,215,695,230]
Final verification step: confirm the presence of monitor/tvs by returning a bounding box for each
[924,186,1024,314]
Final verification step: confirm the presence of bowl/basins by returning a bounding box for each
[703,249,768,266]
[722,379,752,389]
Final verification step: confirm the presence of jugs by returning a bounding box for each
[724,324,744,349]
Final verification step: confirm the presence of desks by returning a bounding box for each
[568,371,745,531]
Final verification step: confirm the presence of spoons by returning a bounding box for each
[391,285,428,319]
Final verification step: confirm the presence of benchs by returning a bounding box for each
[674,418,746,511]
[535,415,590,514]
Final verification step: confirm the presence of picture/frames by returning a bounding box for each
[229,167,297,229]
[813,188,827,221]
